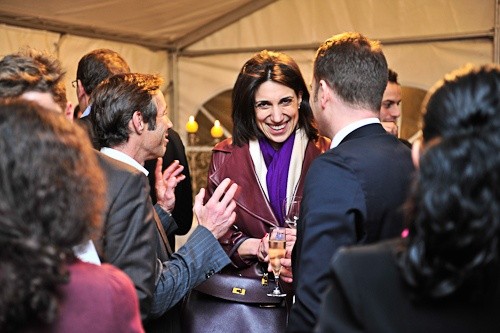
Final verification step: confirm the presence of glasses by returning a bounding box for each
[72,79,78,89]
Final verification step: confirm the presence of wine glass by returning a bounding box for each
[267,228,287,298]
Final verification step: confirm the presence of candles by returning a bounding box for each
[211,120,223,138]
[185,115,198,134]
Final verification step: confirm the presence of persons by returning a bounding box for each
[203,50,331,279]
[0,48,238,333]
[264,31,500,333]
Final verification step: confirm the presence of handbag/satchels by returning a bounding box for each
[183,235,290,333]
